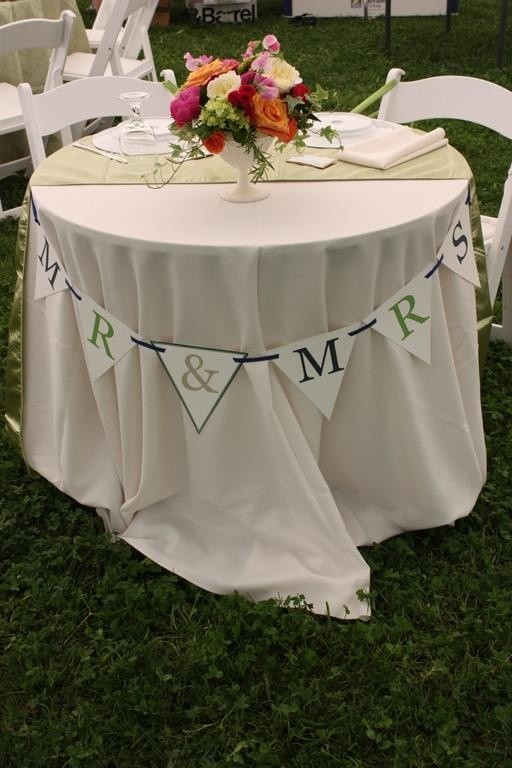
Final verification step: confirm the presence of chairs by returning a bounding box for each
[0,1,158,224]
[378,68,511,345]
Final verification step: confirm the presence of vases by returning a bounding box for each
[218,133,273,204]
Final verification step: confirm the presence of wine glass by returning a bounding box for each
[117,90,160,173]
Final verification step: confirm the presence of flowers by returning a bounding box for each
[140,34,343,190]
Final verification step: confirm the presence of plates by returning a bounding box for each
[306,110,374,137]
[118,116,178,137]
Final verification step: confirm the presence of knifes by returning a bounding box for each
[71,141,127,165]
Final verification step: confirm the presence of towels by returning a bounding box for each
[335,125,448,171]
